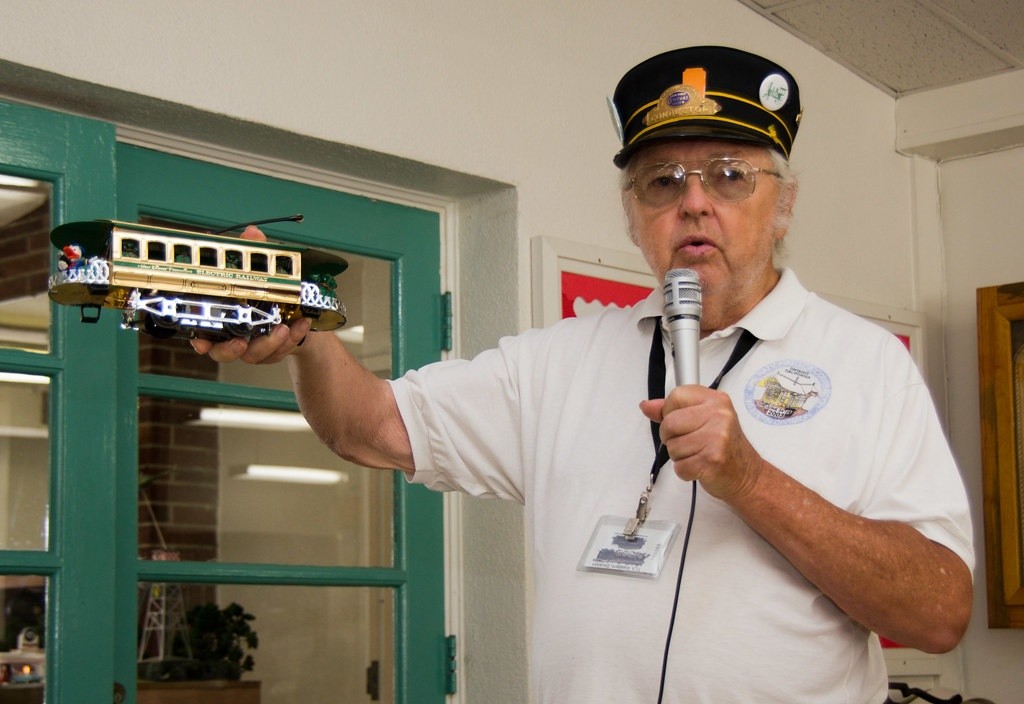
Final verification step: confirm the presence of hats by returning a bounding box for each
[605,45,803,168]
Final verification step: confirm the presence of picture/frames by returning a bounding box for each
[530,236,942,676]
[976,280,1024,631]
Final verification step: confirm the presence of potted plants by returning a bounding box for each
[170,599,259,681]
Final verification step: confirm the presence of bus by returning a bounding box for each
[48,213,349,341]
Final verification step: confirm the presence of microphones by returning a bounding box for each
[664,267,701,386]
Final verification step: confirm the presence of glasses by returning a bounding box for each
[630,156,782,207]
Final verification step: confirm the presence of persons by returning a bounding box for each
[191,44,978,704]
